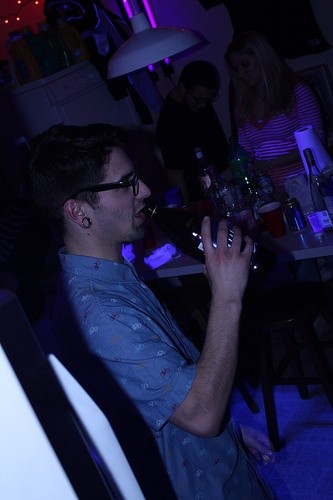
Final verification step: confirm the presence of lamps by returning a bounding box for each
[106,0,202,79]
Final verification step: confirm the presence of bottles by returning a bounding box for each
[303,148,333,232]
[8,29,42,85]
[146,204,260,266]
[194,147,277,240]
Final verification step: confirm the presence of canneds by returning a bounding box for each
[283,197,307,232]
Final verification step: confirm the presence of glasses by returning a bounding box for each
[186,90,221,105]
[72,169,141,200]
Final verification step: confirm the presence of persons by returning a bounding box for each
[153,60,228,201]
[224,27,326,189]
[29,122,275,500]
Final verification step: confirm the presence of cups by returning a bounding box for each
[257,202,285,238]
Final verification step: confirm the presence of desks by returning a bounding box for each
[153,220,333,278]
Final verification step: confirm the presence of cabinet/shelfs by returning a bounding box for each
[0,60,140,151]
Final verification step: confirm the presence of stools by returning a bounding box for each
[162,273,333,453]
